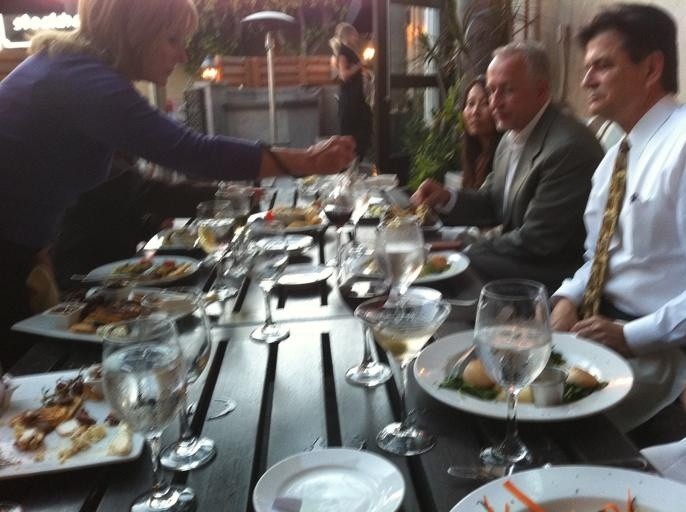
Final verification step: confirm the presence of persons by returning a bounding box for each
[532,6,686,434]
[329,21,377,161]
[53,164,267,297]
[402,75,503,233]
[410,37,605,319]
[2,1,359,376]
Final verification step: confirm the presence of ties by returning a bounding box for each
[578,141,630,321]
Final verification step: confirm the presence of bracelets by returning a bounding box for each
[266,140,307,179]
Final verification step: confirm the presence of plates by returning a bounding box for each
[248,210,330,233]
[84,255,201,285]
[144,216,236,252]
[252,446,406,512]
[256,234,313,256]
[413,325,634,424]
[1,365,146,479]
[351,204,442,231]
[446,466,686,512]
[10,285,198,344]
[273,262,332,289]
[350,250,471,282]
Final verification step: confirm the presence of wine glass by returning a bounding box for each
[100,285,218,512]
[353,294,451,459]
[337,220,425,391]
[320,180,369,265]
[197,199,239,301]
[244,220,291,345]
[474,277,552,471]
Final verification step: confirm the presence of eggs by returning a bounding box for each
[462,362,495,388]
